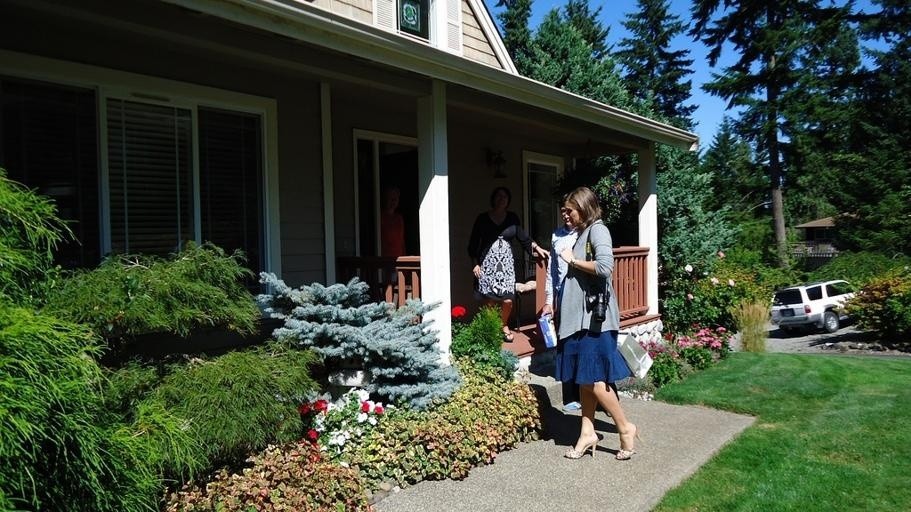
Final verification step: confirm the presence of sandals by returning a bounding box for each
[503,330,515,341]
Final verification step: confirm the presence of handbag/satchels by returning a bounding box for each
[617,333,654,379]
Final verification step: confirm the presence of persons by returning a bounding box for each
[468,185,550,343]
[540,192,620,411]
[382,187,405,286]
[540,185,637,460]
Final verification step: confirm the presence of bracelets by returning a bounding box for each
[570,257,575,268]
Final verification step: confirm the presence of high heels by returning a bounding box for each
[617,423,646,460]
[565,433,601,459]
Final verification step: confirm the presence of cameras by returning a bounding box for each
[585,290,611,321]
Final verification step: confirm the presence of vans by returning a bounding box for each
[768,279,866,334]
